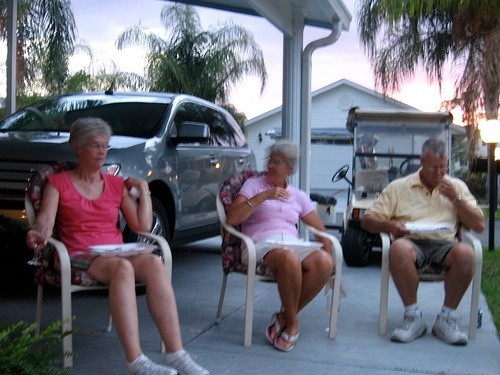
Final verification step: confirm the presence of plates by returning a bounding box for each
[265,238,324,249]
[89,242,158,257]
[404,222,455,234]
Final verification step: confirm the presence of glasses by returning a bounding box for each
[81,142,111,151]
[424,162,449,175]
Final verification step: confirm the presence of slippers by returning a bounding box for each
[266,311,282,344]
[274,328,299,352]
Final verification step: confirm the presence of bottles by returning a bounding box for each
[323,307,331,332]
[477,306,483,328]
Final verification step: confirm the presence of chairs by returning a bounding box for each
[25,161,173,368]
[380,223,483,340]
[216,170,343,347]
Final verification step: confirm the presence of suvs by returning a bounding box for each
[0,89,258,295]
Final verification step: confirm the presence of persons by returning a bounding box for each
[228,140,335,351]
[26,118,210,375]
[360,136,486,345]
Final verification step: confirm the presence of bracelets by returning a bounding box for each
[140,191,151,195]
[246,201,255,209]
[452,195,462,204]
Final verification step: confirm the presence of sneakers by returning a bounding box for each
[390,307,427,343]
[432,314,467,343]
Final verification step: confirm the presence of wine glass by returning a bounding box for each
[27,224,48,266]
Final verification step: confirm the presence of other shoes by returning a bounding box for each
[164,353,210,375]
[126,354,179,375]
